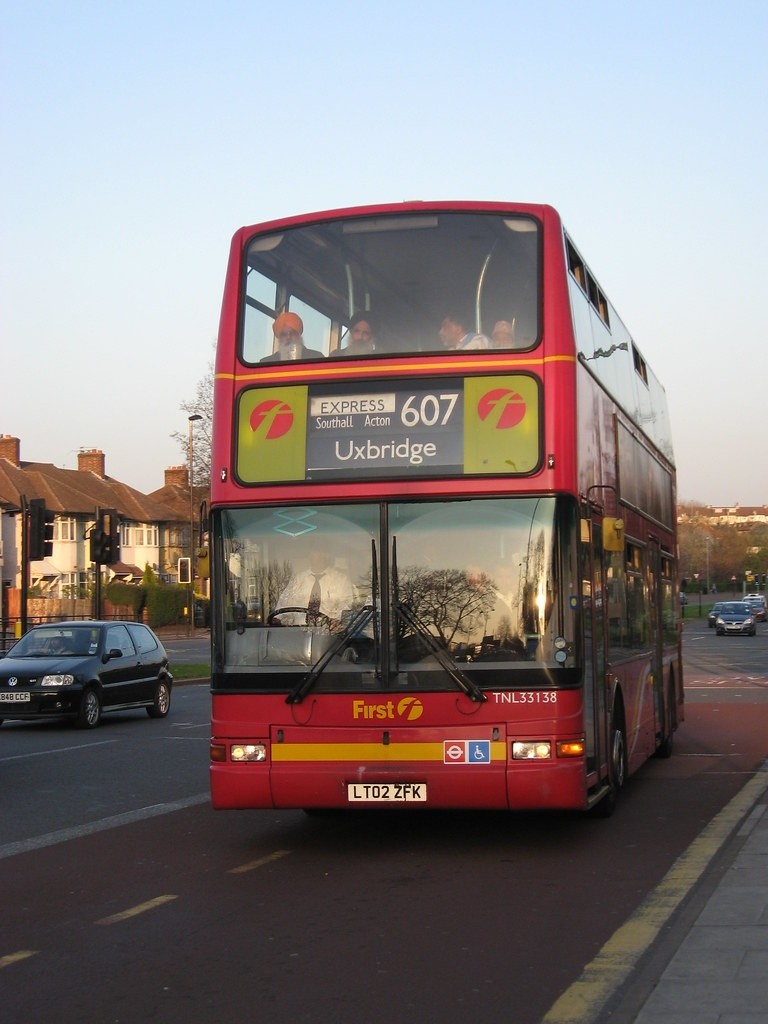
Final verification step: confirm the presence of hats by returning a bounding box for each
[491,321,513,337]
[273,312,302,337]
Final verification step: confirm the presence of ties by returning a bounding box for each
[306,573,326,625]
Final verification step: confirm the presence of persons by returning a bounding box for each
[330,311,377,357]
[40,637,74,654]
[439,310,512,350]
[272,538,353,632]
[260,313,324,362]
[447,557,515,661]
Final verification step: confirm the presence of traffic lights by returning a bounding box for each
[762,574,766,586]
[755,575,758,584]
[29,499,54,560]
[178,556,191,583]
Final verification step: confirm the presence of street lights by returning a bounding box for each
[189,414,202,632]
[694,573,701,616]
[90,528,107,564]
[731,576,736,599]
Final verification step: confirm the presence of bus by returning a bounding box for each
[195,200,685,826]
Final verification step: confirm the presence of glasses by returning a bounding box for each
[350,327,369,337]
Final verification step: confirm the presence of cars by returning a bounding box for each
[0,620,174,729]
[716,601,757,637]
[680,592,688,605]
[742,593,768,622]
[707,602,725,628]
